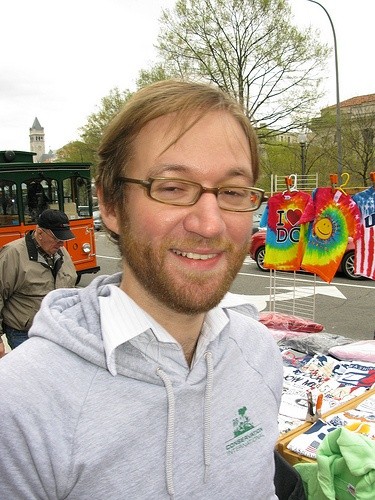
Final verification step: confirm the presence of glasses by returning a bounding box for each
[116,176,269,212]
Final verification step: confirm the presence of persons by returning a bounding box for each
[0,209,77,358]
[0,178,71,217]
[0,77,284,500]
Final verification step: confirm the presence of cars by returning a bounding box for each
[249,227,372,281]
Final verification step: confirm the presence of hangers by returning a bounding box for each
[282,174,299,196]
[334,172,350,196]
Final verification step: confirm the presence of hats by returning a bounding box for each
[38,209,77,241]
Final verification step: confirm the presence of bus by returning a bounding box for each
[0,150,101,285]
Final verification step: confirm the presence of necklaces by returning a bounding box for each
[44,251,55,268]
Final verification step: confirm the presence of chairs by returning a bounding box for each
[48,196,77,217]
